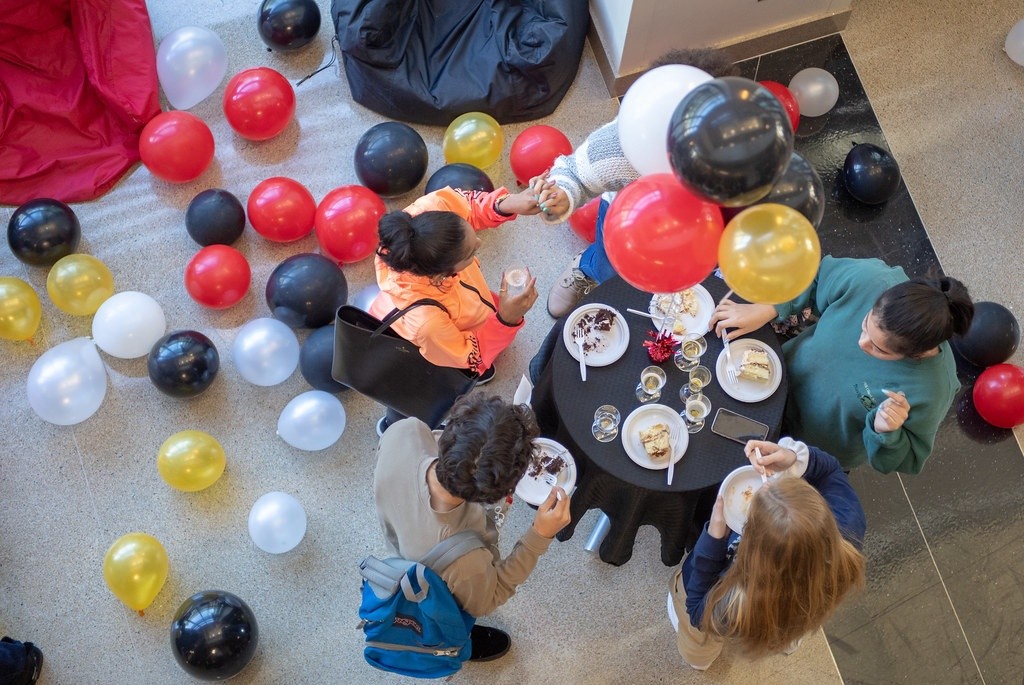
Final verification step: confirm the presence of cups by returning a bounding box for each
[504,264,527,297]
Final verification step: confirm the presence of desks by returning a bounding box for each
[527,273,790,566]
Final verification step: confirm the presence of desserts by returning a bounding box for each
[593,309,614,331]
[735,348,770,384]
[673,288,696,337]
[639,423,671,459]
[527,456,564,477]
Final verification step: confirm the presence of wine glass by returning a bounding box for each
[679,394,712,434]
[591,404,621,443]
[679,366,712,404]
[674,333,707,372]
[635,365,667,405]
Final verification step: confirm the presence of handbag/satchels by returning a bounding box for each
[331,298,479,430]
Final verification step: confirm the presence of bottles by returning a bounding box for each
[514,437,577,506]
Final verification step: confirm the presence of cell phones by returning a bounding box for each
[711,408,770,445]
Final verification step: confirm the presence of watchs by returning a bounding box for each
[495,193,510,212]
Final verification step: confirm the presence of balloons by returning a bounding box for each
[953,302,1019,364]
[974,363,1024,428]
[1002,19,1024,66]
[249,492,307,553]
[171,590,258,680]
[158,431,225,491]
[157,26,227,111]
[104,534,167,616]
[2,64,900,454]
[257,0,321,52]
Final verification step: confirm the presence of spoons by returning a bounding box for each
[625,307,674,323]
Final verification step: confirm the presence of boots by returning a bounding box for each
[547,249,597,319]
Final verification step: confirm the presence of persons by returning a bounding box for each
[375,168,557,437]
[668,436,866,671]
[708,254,973,476]
[374,392,571,662]
[529,46,741,318]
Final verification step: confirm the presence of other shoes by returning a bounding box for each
[375,416,446,437]
[667,593,711,670]
[476,363,496,386]
[468,624,510,662]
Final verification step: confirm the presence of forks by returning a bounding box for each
[574,328,586,381]
[667,424,680,485]
[721,328,738,384]
[546,474,562,502]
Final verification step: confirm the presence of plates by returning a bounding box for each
[715,338,783,403]
[562,303,630,368]
[650,284,715,342]
[621,403,689,470]
[717,465,775,536]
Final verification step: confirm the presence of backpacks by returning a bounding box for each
[354,531,487,679]
